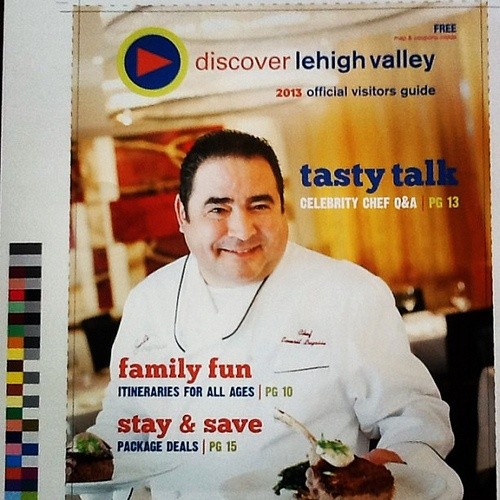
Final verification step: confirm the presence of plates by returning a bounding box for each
[64,456,180,495]
[215,442,465,500]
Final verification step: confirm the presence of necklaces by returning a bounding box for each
[171,251,272,354]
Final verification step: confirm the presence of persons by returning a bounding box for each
[81,129,456,499]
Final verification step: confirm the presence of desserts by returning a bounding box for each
[66,426,115,483]
[301,438,396,500]
